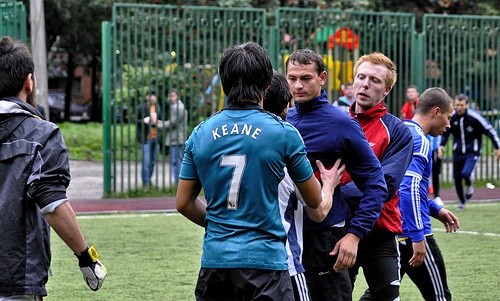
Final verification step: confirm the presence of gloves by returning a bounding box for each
[74,247,107,292]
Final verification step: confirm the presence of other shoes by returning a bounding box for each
[457,202,465,212]
[466,184,473,200]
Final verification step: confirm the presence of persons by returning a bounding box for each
[286,50,388,301]
[438,93,500,209]
[136,89,161,192]
[426,135,444,204]
[175,42,322,301]
[369,52,414,301]
[0,38,107,301]
[158,90,187,186]
[362,87,460,301]
[333,84,356,108]
[400,86,419,121]
[263,76,345,301]
